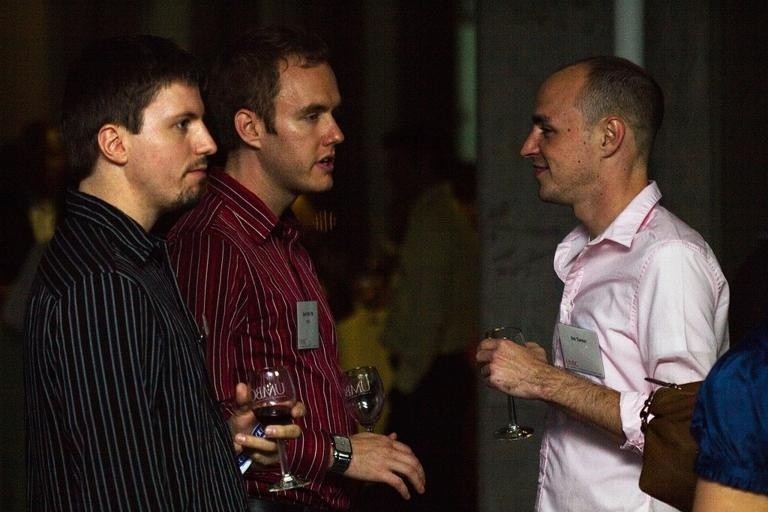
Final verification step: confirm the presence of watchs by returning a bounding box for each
[330,433,353,475]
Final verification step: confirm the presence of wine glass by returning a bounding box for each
[249,366,314,494]
[483,326,537,442]
[339,364,384,433]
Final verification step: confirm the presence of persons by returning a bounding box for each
[22,34,309,512]
[475,54,733,512]
[167,28,430,512]
[692,323,767,512]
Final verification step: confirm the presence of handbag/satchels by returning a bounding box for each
[638,380,702,512]
